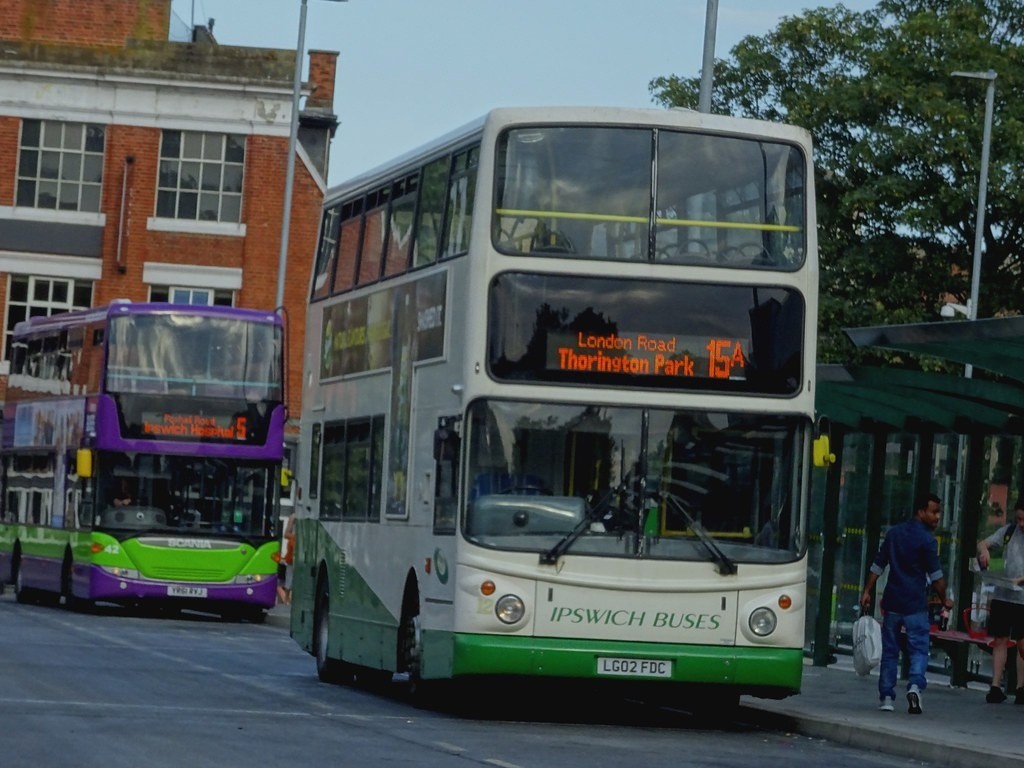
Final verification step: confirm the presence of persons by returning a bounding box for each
[470,445,542,498]
[113,480,131,507]
[861,493,953,712]
[977,503,1024,705]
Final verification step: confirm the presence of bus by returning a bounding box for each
[286,104,837,711]
[0,296,294,624]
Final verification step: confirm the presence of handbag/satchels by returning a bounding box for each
[852,606,882,675]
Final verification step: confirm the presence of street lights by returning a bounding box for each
[276,0,349,320]
[948,67,997,631]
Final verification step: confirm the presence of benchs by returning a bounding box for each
[931,630,995,688]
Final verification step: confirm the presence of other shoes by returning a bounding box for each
[986,687,1007,704]
[1014,687,1024,706]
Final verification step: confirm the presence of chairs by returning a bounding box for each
[498,225,776,265]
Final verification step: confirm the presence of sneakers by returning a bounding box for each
[879,696,896,712]
[906,684,922,714]
